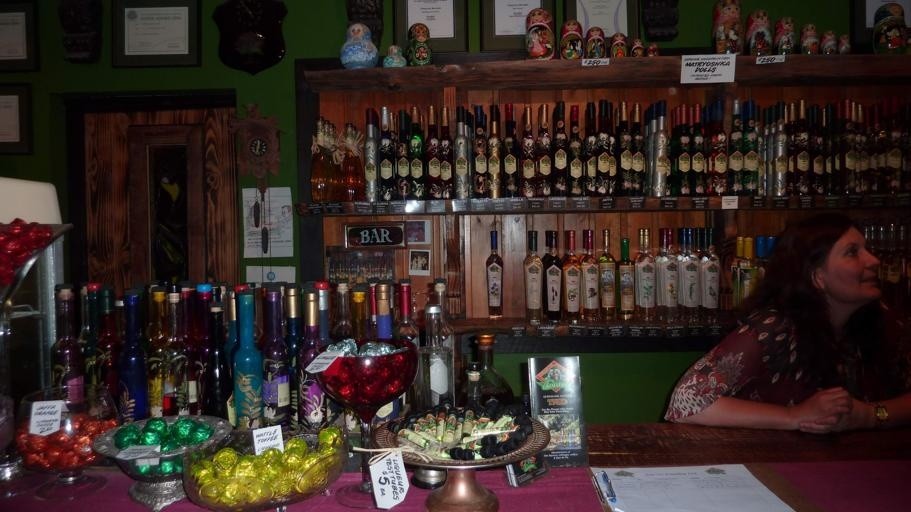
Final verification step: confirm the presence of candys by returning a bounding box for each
[189,426,343,506]
[388,400,532,460]
[0,218,53,284]
[114,418,213,475]
[16,413,119,469]
[322,339,406,404]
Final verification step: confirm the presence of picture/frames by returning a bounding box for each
[560,1,640,45]
[390,0,470,51]
[108,1,202,70]
[0,1,41,73]
[0,82,34,156]
[476,0,554,53]
[849,1,911,50]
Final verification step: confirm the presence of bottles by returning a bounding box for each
[486,229,505,316]
[526,223,911,330]
[49,277,455,417]
[458,335,514,404]
[311,98,911,202]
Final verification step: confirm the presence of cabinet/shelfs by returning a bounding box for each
[292,49,911,355]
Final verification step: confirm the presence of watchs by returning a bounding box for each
[869,397,892,427]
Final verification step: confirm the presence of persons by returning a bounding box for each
[662,212,911,434]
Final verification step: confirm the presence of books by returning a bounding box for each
[584,459,810,512]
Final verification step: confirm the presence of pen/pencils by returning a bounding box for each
[601,472,617,503]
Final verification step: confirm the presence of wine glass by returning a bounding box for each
[314,340,418,508]
[18,382,120,501]
